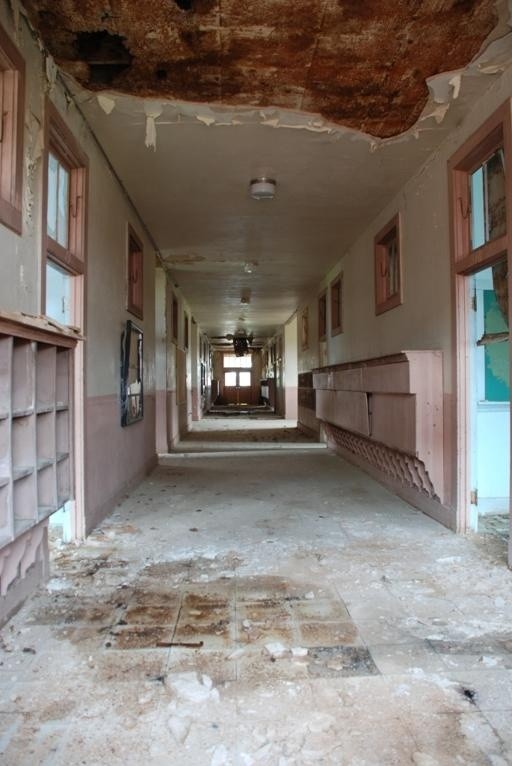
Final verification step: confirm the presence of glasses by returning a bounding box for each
[246,177,276,201]
[240,262,257,274]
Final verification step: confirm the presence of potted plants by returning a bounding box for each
[121,320,144,427]
